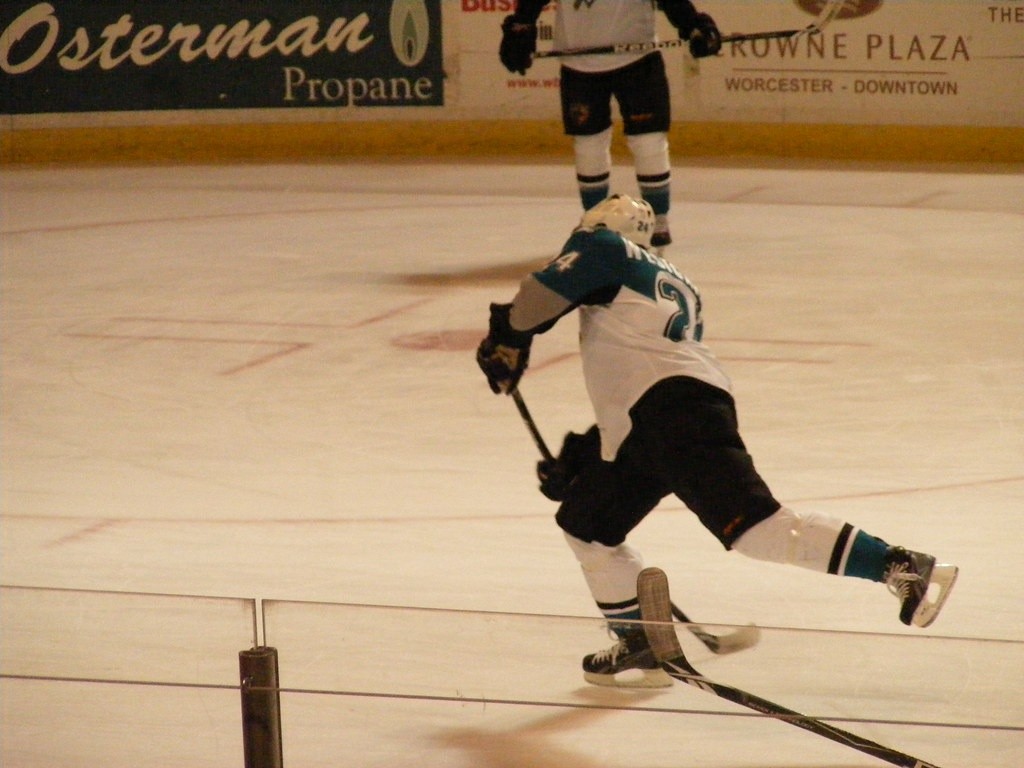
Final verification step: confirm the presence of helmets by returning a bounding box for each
[570,193,656,250]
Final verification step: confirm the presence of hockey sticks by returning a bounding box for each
[528,0,842,60]
[509,386,763,657]
[636,567,946,768]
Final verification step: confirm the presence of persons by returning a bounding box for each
[477,194,957,688]
[499,0,721,246]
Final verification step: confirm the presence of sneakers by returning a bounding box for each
[872,536,959,627]
[651,212,672,255]
[580,599,673,687]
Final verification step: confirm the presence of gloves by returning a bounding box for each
[477,302,534,395]
[676,14,722,59]
[536,424,601,500]
[499,13,538,76]
